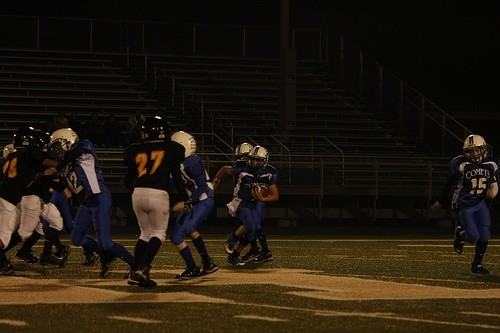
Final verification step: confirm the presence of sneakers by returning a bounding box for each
[200,261,217,276]
[224,243,240,256]
[122,265,134,278]
[227,256,245,266]
[242,248,261,262]
[454,225,466,254]
[100,255,117,276]
[39,254,64,266]
[15,250,39,263]
[55,245,71,267]
[81,252,98,266]
[0,260,15,275]
[253,250,273,263]
[469,263,490,277]
[128,269,157,286]
[180,265,202,279]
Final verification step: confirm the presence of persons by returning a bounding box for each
[0,126,134,277]
[171,131,219,280]
[52,110,169,146]
[214,145,279,262]
[431,134,499,273]
[127,116,185,286]
[233,143,273,261]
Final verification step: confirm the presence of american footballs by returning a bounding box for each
[254,182,271,198]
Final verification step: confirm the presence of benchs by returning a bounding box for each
[0,49,451,186]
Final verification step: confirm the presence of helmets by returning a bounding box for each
[246,145,269,167]
[34,128,50,151]
[462,134,487,163]
[144,115,166,140]
[235,142,254,161]
[3,144,17,158]
[171,130,196,158]
[14,125,38,150]
[47,126,80,162]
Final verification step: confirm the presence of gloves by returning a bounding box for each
[183,201,193,219]
